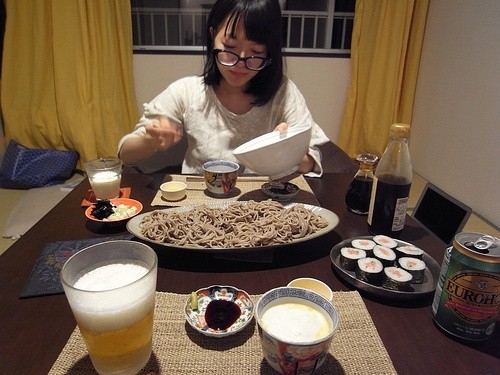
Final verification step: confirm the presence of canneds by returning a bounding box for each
[431,232,500,343]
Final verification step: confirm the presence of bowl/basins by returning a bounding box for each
[287,277,334,303]
[160,181,188,203]
[232,124,312,181]
[254,286,339,375]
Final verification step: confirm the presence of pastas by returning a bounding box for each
[137,198,329,248]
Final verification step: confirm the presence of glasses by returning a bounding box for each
[212,31,272,71]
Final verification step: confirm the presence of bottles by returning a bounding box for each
[366,121,413,237]
[344,153,379,216]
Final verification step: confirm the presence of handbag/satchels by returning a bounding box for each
[0,139,80,190]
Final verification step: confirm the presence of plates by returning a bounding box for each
[125,199,340,253]
[84,197,144,223]
[184,285,254,338]
[262,181,300,201]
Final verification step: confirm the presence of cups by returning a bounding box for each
[201,161,240,198]
[84,158,123,201]
[59,240,159,375]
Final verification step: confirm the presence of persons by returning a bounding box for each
[116,0,327,177]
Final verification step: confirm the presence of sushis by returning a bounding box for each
[340,234,426,290]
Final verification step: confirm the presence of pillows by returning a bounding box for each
[0,139,80,189]
